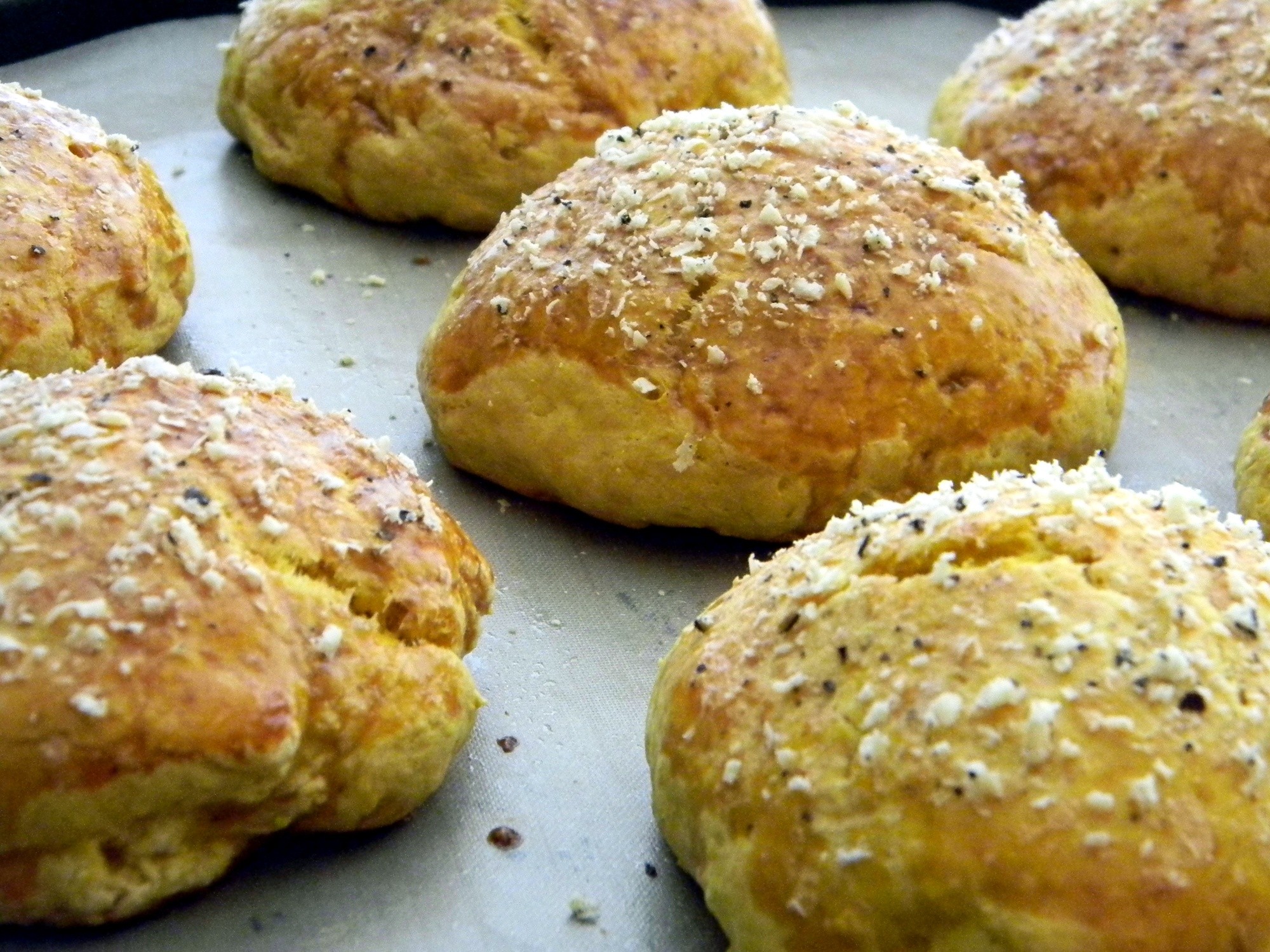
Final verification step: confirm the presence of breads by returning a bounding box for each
[1,0,1269,952]
